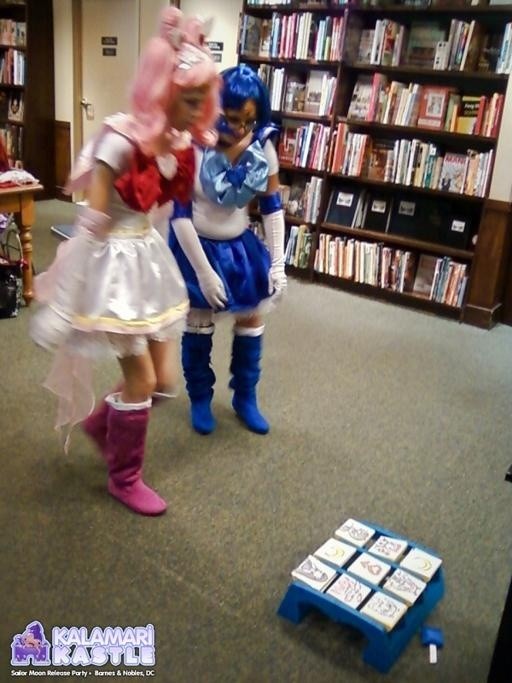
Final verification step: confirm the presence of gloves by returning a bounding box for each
[27,205,113,355]
[255,191,289,305]
[167,197,229,311]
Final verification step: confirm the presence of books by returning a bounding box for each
[1,18,41,188]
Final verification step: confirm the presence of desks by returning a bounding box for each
[0,184,46,308]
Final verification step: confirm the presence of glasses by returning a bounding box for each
[224,118,259,133]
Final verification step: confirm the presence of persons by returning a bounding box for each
[26,4,226,515]
[167,64,285,434]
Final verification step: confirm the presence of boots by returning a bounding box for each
[79,380,181,518]
[180,322,218,436]
[227,322,270,435]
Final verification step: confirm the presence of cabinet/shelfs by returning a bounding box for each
[236,1,511,320]
[0,0,57,203]
